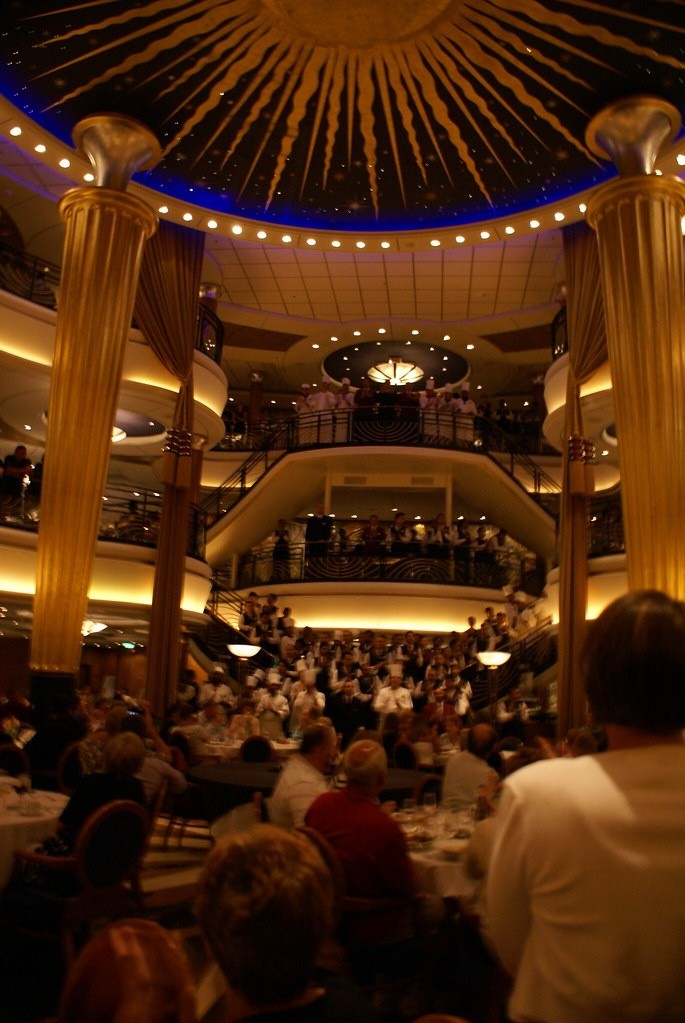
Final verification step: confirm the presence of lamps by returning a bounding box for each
[228,643,261,713]
[368,357,423,386]
[112,428,128,443]
[477,650,511,726]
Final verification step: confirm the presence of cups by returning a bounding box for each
[422,792,437,813]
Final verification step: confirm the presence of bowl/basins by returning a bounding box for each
[432,839,470,861]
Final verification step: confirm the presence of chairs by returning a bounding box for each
[0,732,522,1023]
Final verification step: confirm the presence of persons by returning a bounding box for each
[116,501,509,580]
[0,444,46,521]
[0,584,685,1023]
[232,375,540,452]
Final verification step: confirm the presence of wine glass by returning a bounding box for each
[401,800,418,832]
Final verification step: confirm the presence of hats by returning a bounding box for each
[322,376,329,384]
[503,584,513,597]
[302,384,309,388]
[427,380,434,389]
[461,382,470,391]
[514,591,527,603]
[394,647,404,660]
[444,383,453,393]
[214,659,316,684]
[388,665,403,677]
[342,378,350,385]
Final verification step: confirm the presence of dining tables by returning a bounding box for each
[0,790,70,887]
[193,736,303,762]
[389,808,487,900]
